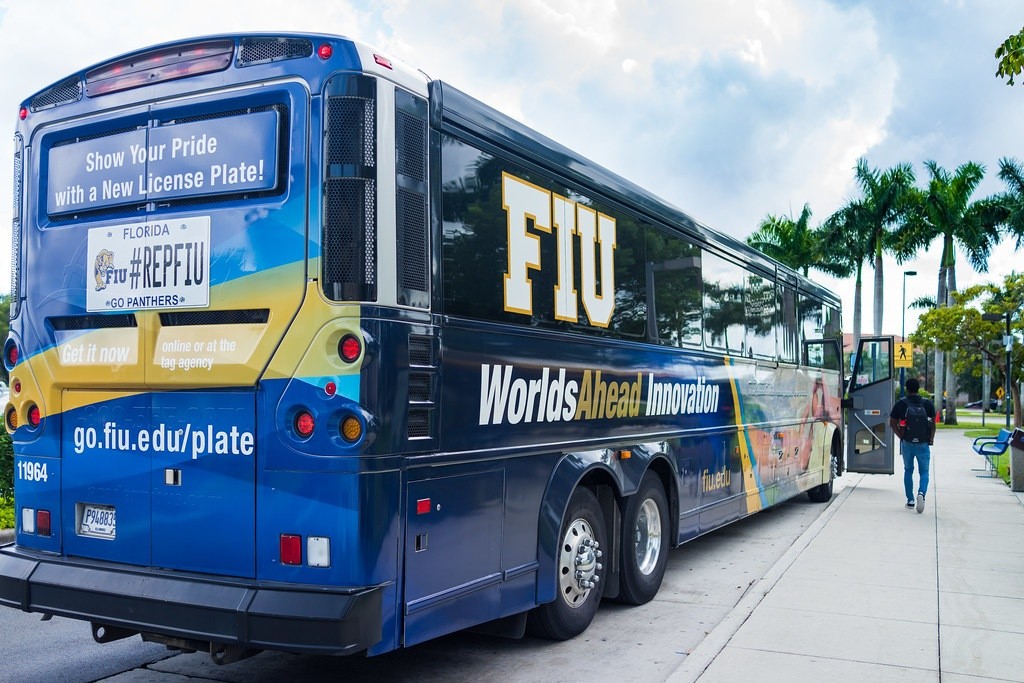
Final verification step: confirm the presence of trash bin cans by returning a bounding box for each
[1007,427,1024,492]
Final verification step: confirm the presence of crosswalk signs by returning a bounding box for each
[894,343,914,368]
[997,387,1005,398]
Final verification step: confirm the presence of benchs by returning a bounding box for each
[971,428,1012,478]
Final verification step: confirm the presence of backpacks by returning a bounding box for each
[901,396,931,443]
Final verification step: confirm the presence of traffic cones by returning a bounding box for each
[934,411,941,423]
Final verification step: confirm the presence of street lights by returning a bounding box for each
[981,313,1012,432]
[901,269,918,345]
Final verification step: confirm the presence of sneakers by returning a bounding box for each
[917,492,926,513]
[905,500,915,509]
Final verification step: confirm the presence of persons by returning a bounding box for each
[889,378,937,513]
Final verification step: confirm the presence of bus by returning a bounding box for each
[0,30,895,659]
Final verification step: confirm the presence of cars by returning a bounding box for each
[965,399,998,409]
